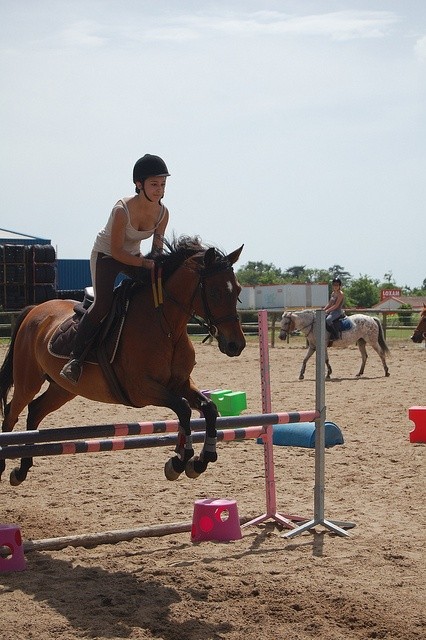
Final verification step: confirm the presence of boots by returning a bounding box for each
[63,312,102,383]
[329,325,339,341]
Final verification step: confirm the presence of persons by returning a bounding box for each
[321,277,346,342]
[58,152,170,385]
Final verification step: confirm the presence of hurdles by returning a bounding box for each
[0,310,356,554]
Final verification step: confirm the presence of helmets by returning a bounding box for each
[333,278,341,286]
[133,154,171,183]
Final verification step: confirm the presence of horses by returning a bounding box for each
[277,307,393,380]
[0,229,248,488]
[410,301,426,344]
